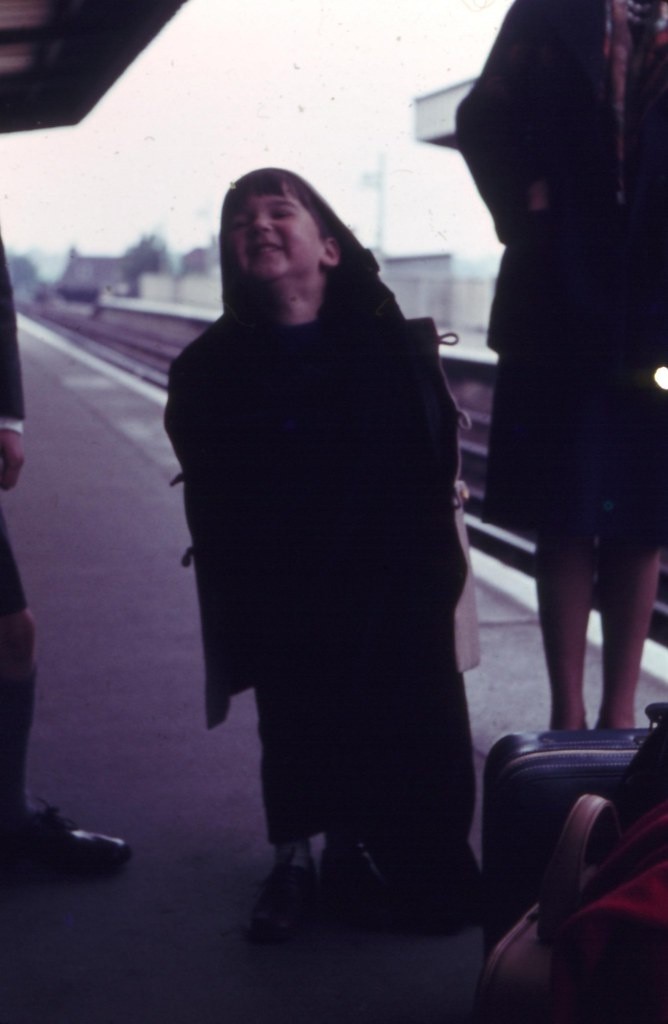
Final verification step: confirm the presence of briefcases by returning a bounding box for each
[473,726,662,968]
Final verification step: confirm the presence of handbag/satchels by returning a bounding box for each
[462,793,626,1023]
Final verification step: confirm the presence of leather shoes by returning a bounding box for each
[0,808,130,874]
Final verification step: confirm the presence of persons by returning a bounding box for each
[164,167,483,944]
[454,0,668,735]
[0,235,135,867]
